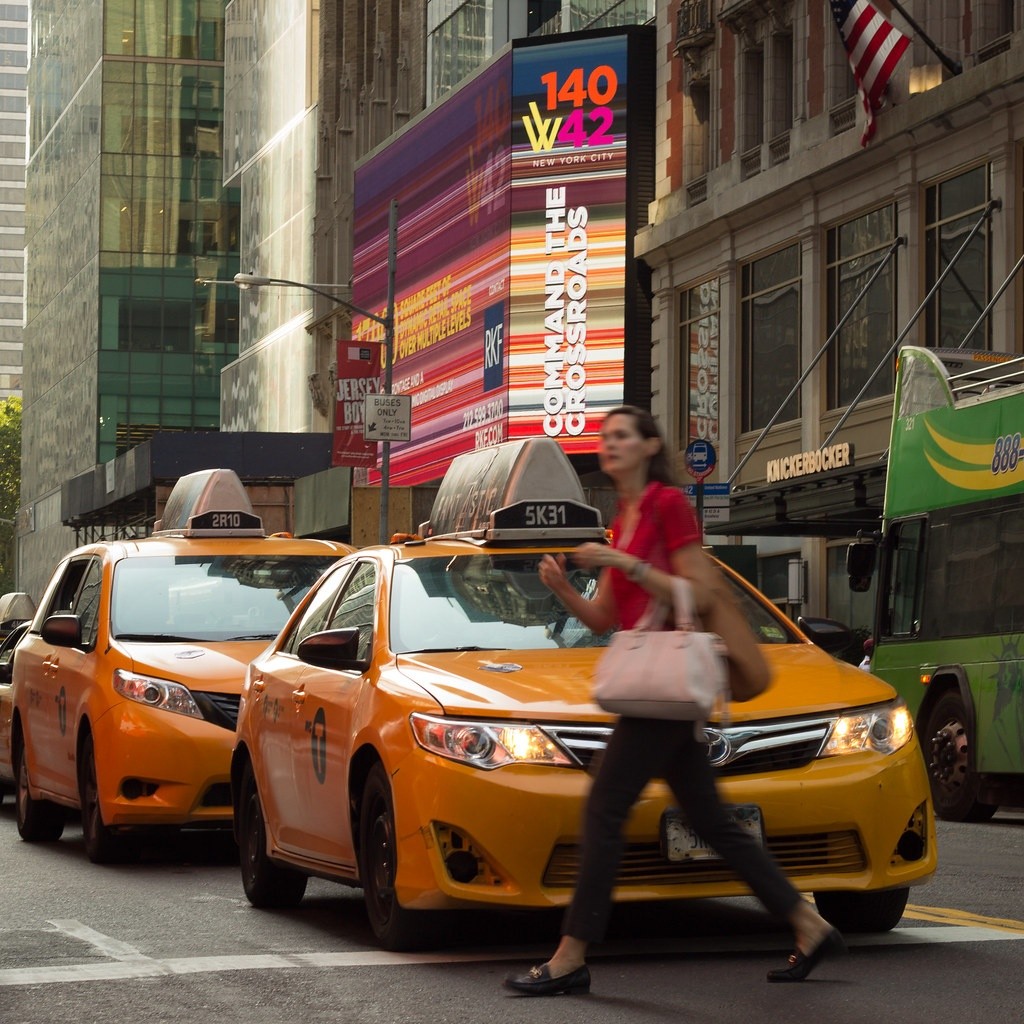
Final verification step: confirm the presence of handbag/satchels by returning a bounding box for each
[592,575,723,721]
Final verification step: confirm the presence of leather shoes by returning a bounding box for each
[767,928,845,982]
[508,963,591,996]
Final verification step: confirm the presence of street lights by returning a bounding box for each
[233,196,399,547]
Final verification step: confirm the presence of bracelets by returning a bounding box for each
[630,560,651,584]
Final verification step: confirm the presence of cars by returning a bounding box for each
[6,469,365,860]
[220,436,938,957]
[0,592,36,802]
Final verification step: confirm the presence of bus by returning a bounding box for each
[845,343,1024,825]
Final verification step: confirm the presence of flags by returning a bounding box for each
[830,0,912,147]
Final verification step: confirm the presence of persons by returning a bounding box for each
[505,406,842,992]
